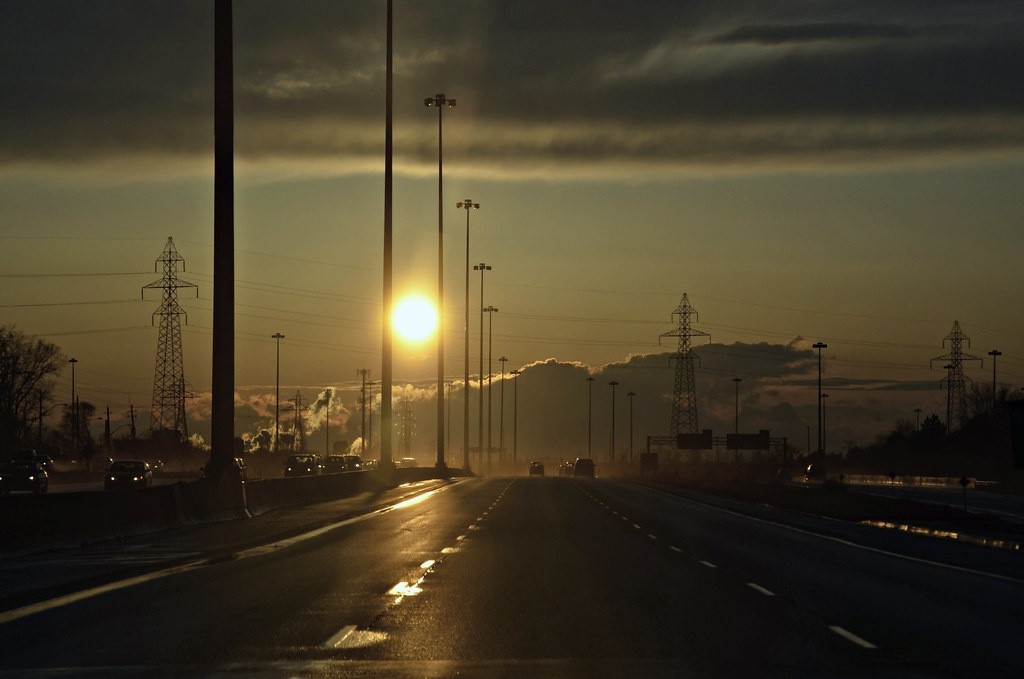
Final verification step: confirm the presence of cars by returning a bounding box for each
[104,458,164,489]
[0,448,53,495]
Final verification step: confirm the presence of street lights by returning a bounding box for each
[69,358,78,445]
[586,376,595,458]
[812,342,829,453]
[424,92,457,478]
[943,365,954,432]
[627,391,637,462]
[472,263,521,472]
[272,332,285,450]
[609,380,619,464]
[913,408,922,431]
[455,197,479,473]
[366,381,376,449]
[732,378,741,461]
[988,349,1002,404]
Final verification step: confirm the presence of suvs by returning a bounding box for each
[529,462,544,476]
[200,458,247,482]
[284,453,416,476]
[559,458,594,478]
